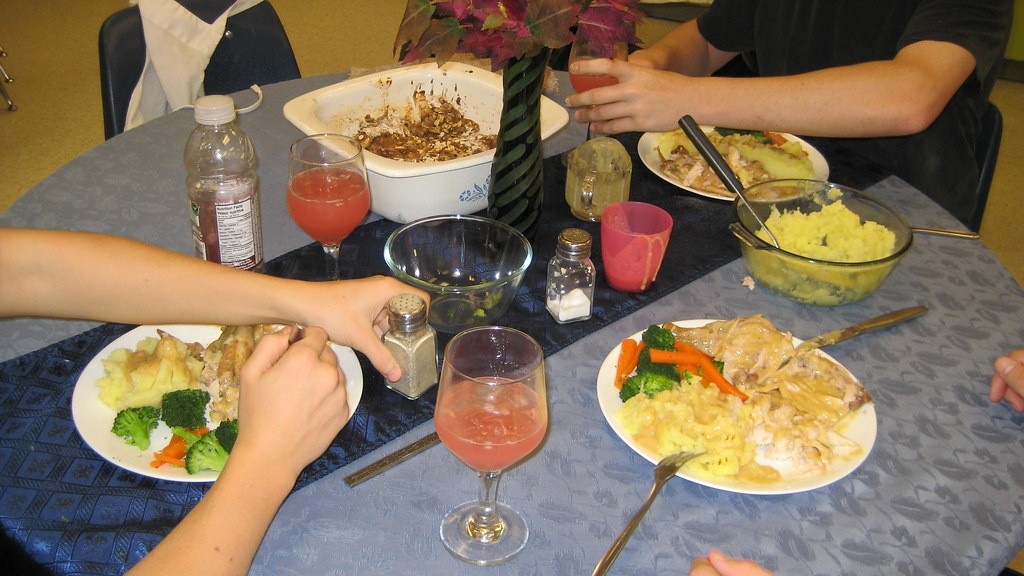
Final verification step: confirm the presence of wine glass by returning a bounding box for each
[286,132,371,281]
[432,326,548,564]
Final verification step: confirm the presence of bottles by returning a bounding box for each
[184,94,265,277]
[544,227,596,325]
[380,293,439,400]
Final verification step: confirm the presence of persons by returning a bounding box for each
[691,348,1023,575]
[0,226,430,576]
[566,1,1016,223]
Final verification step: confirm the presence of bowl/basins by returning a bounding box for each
[729,178,912,307]
[282,60,570,226]
[383,213,533,335]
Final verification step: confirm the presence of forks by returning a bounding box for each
[592,450,708,576]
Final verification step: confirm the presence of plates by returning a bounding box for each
[72,322,364,483]
[596,319,877,495]
[637,123,830,202]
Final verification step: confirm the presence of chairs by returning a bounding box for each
[98,0,303,141]
[709,53,1003,232]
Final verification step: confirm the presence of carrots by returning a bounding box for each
[150,426,210,468]
[767,131,808,157]
[615,339,749,402]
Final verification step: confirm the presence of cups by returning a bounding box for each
[569,28,629,109]
[600,202,673,293]
[566,136,632,222]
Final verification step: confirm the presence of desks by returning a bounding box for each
[0,58,1024,575]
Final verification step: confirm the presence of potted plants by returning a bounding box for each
[392,0,647,246]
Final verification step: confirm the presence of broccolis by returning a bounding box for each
[186,419,238,475]
[618,325,682,402]
[114,390,210,450]
[714,127,770,143]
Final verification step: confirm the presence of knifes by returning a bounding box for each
[794,305,929,354]
